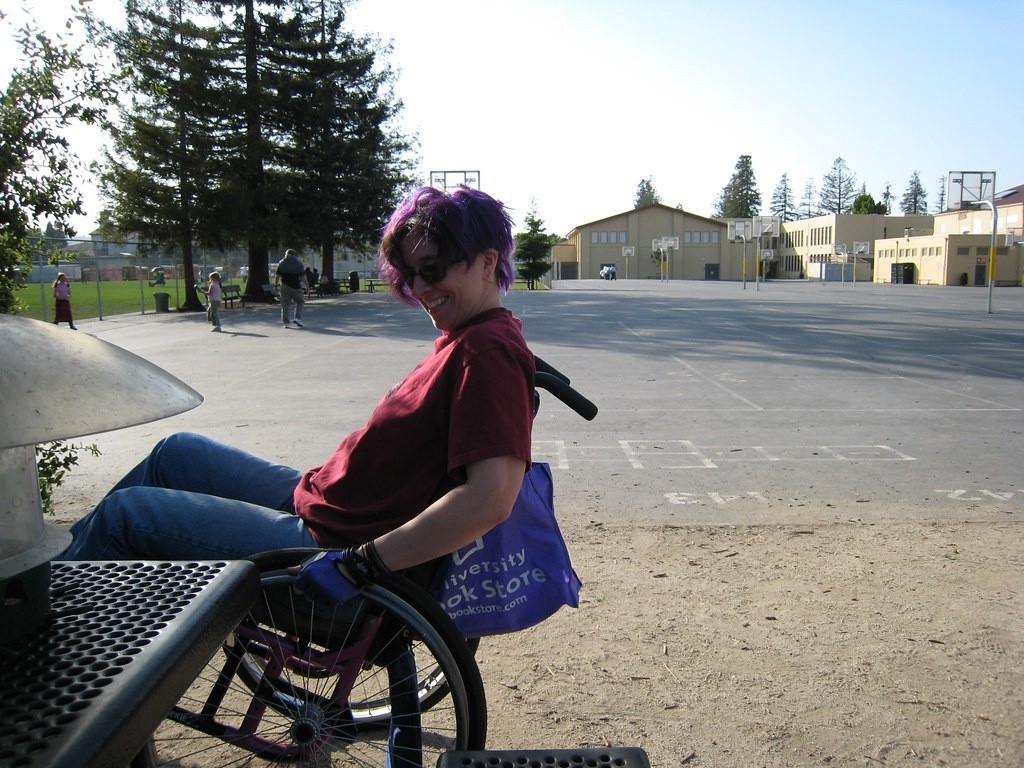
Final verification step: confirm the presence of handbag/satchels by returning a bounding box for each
[71,326,77,330]
[412,463,582,638]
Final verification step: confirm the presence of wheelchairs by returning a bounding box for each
[133,356,597,768]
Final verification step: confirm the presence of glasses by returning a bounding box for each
[405,257,466,289]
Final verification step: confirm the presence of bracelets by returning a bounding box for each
[360,541,393,579]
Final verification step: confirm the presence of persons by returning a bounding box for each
[149,273,166,287]
[610,264,617,280]
[305,266,314,287]
[275,249,309,328]
[241,264,248,282]
[53,272,77,330]
[195,271,223,332]
[52,185,537,605]
[603,264,610,280]
[314,266,318,284]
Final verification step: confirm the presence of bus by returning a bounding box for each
[122,262,200,281]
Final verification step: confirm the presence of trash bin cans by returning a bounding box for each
[961,273,968,285]
[349,271,359,293]
[153,292,170,312]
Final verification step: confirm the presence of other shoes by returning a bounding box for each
[285,324,291,328]
[211,327,221,332]
[293,318,303,327]
